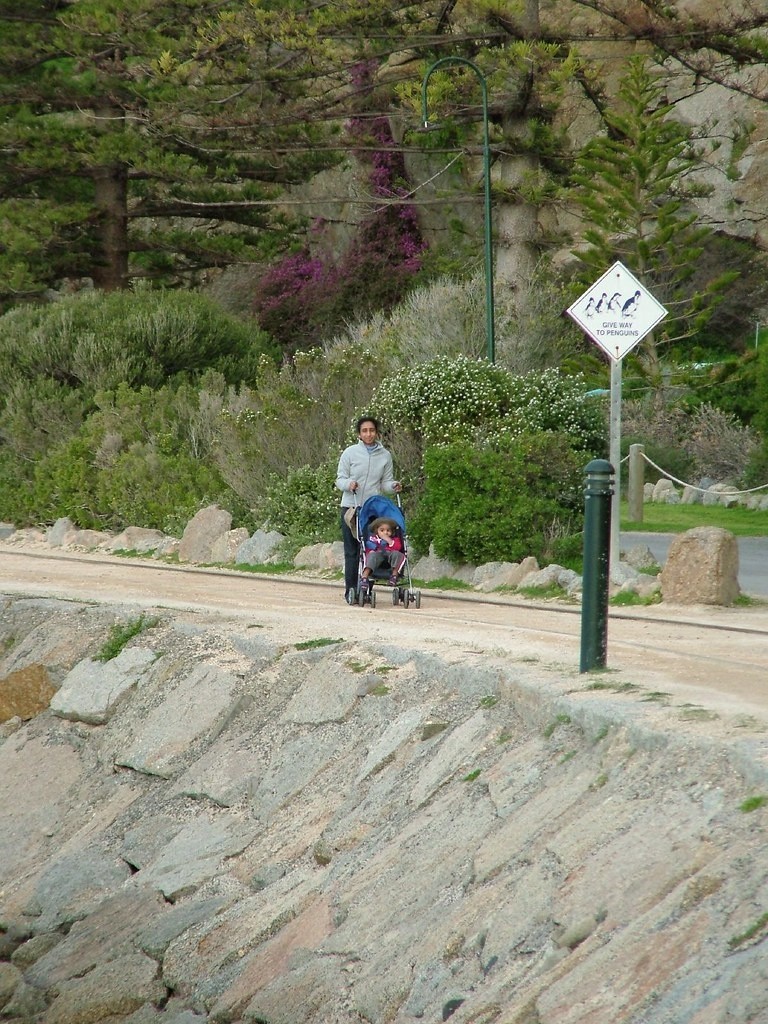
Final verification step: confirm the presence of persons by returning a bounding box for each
[335,417,404,604]
[359,517,406,591]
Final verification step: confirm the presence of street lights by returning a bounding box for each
[417,54,504,371]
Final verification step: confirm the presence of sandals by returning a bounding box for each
[359,577,369,591]
[387,575,397,586]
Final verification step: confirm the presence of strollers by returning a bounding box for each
[347,482,421,609]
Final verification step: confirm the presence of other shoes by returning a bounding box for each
[363,596,371,603]
[346,596,358,604]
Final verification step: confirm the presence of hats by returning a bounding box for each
[344,506,358,540]
[369,517,398,536]
